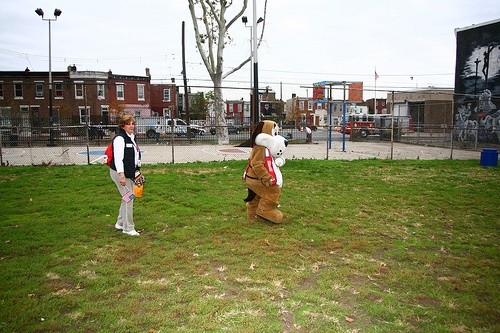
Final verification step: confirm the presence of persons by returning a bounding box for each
[155,122,162,145]
[90,122,115,139]
[109,115,143,236]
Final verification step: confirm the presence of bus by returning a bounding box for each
[340,114,392,137]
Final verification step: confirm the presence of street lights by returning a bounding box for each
[242,16,264,139]
[34,7,62,147]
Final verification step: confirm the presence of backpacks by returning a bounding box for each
[103,141,113,166]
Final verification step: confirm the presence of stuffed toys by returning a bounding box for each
[243,120,289,223]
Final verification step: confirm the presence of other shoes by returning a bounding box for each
[123,229,140,236]
[115,223,123,230]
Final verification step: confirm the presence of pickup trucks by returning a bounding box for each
[146,119,198,139]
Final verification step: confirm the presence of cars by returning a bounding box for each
[299,124,317,131]
[241,122,257,131]
[210,123,240,135]
[192,127,206,135]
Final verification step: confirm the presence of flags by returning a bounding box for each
[375,72,379,80]
